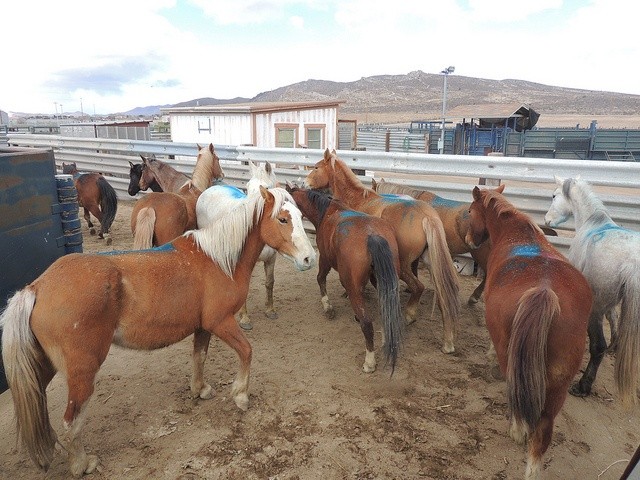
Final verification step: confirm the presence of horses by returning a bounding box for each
[62,161,119,246]
[138,153,191,193]
[0,185,317,479]
[285,183,409,382]
[543,172,640,415]
[130,142,227,250]
[195,157,281,330]
[465,183,593,480]
[127,161,164,196]
[302,148,465,354]
[372,177,488,305]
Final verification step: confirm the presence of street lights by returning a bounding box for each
[440,67,457,156]
